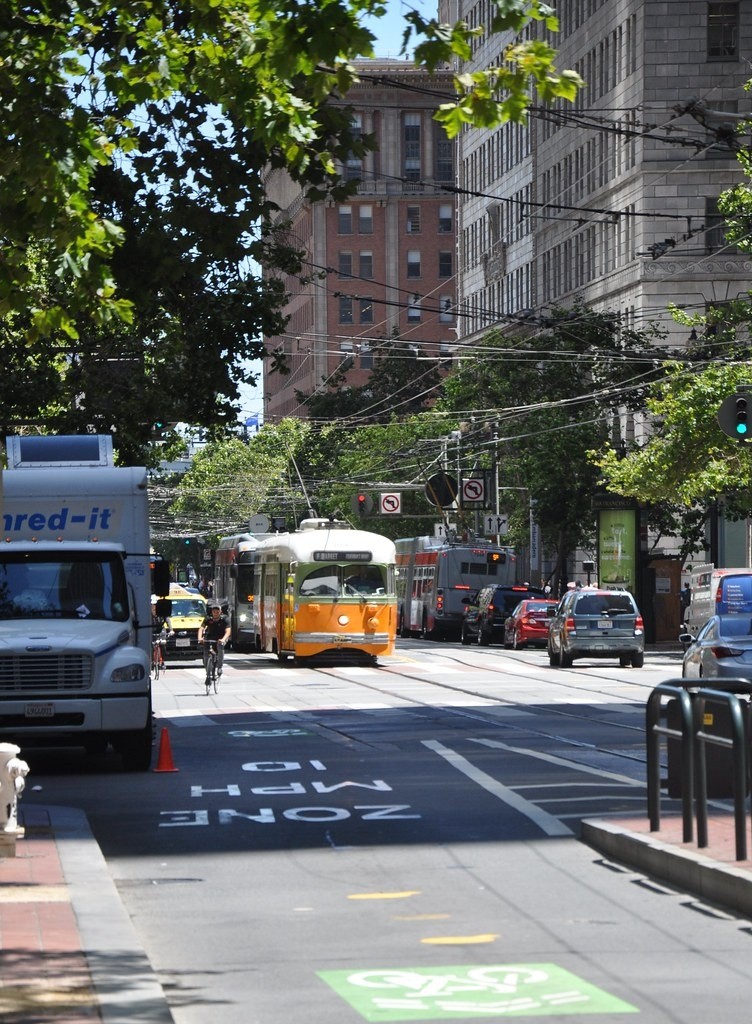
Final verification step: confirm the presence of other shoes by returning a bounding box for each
[205,678,211,685]
[217,668,222,675]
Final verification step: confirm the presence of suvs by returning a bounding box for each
[547,588,645,668]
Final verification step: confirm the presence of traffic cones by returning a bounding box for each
[153,728,179,772]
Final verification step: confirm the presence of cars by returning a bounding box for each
[172,588,204,615]
[504,599,561,650]
[461,584,548,646]
[156,589,208,665]
[679,614,752,696]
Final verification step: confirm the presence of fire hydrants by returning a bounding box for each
[0,742,29,839]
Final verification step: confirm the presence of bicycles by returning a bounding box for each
[198,640,222,696]
[152,633,171,680]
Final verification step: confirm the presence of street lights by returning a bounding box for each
[542,541,559,554]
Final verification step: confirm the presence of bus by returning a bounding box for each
[252,519,398,664]
[212,532,290,653]
[394,536,516,641]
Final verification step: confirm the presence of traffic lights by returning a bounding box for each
[583,561,594,572]
[185,540,190,545]
[272,517,285,530]
[736,398,748,434]
[359,496,365,514]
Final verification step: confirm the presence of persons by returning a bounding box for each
[152,604,175,669]
[198,606,231,675]
[541,578,598,593]
[681,582,691,599]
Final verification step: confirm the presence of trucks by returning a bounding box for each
[0,434,171,771]
[680,563,752,654]
